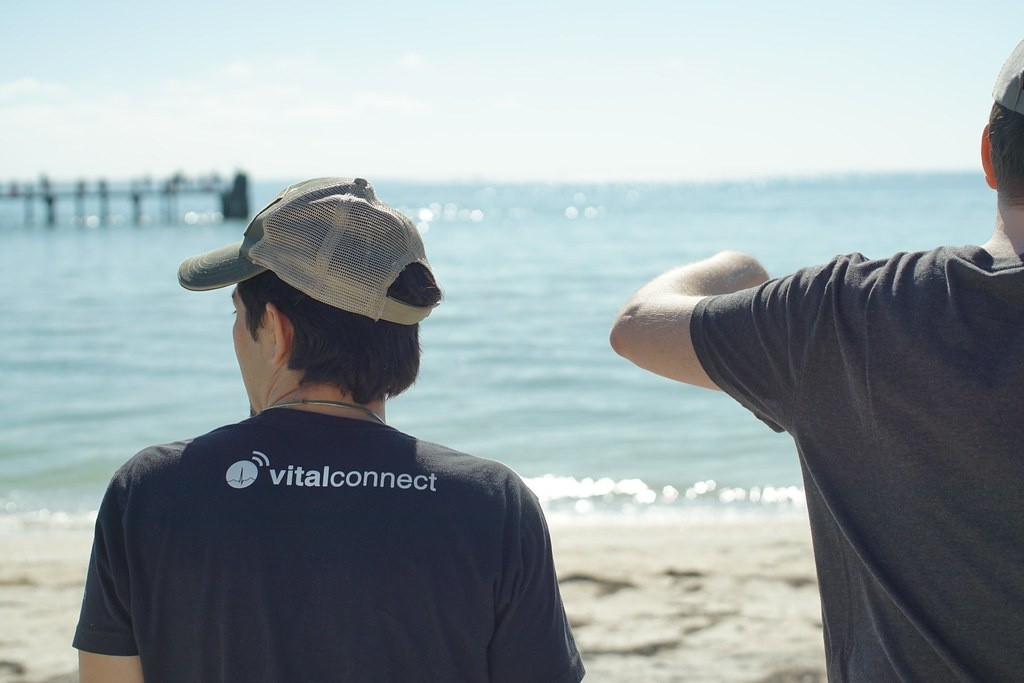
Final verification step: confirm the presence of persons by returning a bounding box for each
[610,40,1024,683]
[71,176,587,683]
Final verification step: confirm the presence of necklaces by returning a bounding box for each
[250,400,386,426]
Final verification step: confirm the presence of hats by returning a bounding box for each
[992,38,1024,115]
[178,178,440,325]
